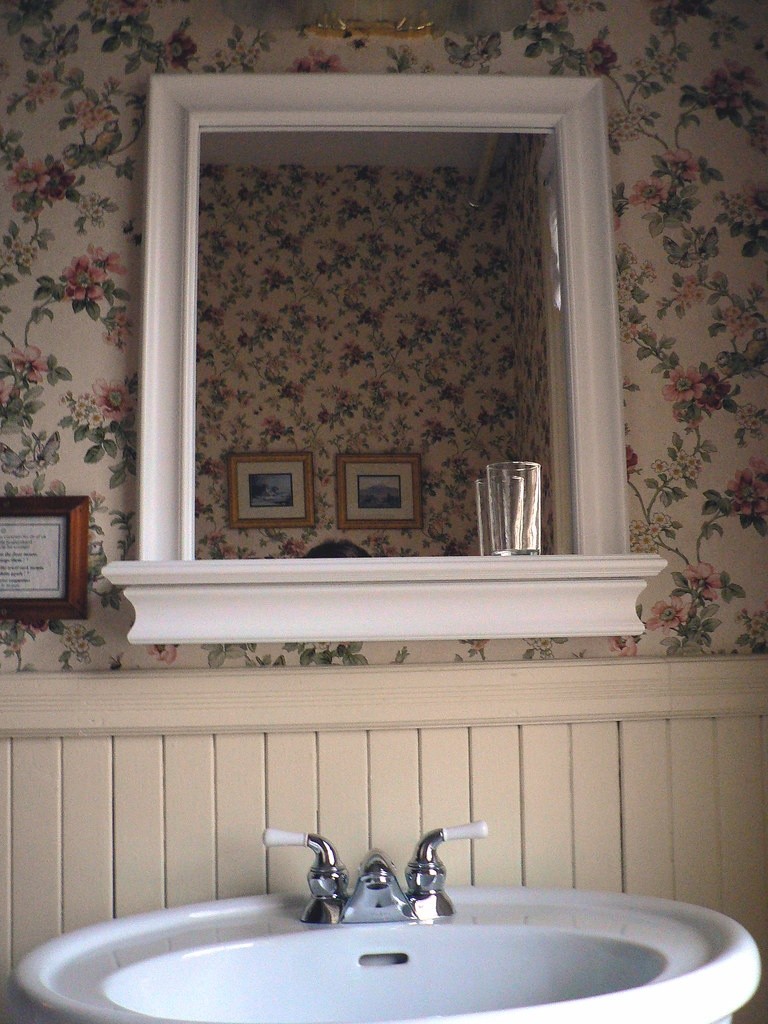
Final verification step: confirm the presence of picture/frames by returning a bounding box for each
[227,451,315,528]
[336,452,423,530]
[0,495,88,620]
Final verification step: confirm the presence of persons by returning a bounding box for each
[303,538,372,559]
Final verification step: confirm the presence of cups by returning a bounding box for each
[476,476,527,556]
[487,460,542,557]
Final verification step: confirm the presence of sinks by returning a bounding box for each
[14,891,760,1024]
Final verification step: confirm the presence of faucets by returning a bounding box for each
[264,819,489,924]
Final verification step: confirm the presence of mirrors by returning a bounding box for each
[136,72,629,560]
